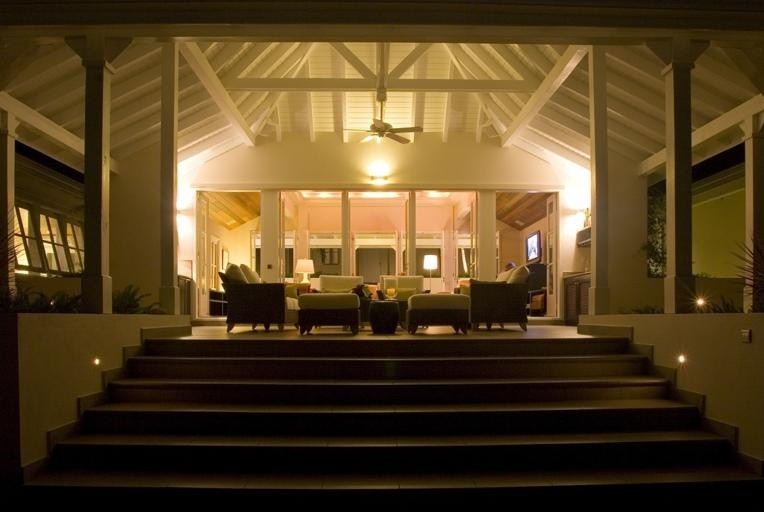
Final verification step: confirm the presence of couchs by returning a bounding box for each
[297,293,361,334]
[406,294,470,335]
[218,265,299,334]
[319,274,373,322]
[452,265,529,334]
[377,274,432,322]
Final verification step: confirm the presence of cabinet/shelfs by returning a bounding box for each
[563,274,591,326]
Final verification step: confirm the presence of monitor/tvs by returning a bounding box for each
[527,231,540,261]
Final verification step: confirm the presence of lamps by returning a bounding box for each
[423,254,438,290]
[294,259,315,284]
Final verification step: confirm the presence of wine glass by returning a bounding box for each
[382,278,399,301]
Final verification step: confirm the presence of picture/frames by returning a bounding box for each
[525,231,541,262]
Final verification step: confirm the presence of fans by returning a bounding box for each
[343,102,423,144]
